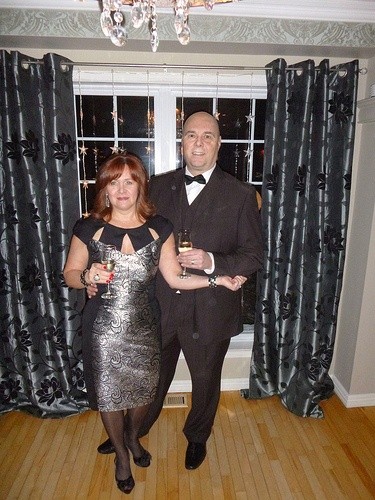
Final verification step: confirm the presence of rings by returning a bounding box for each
[94,274,100,281]
[191,260,195,265]
[239,286,241,288]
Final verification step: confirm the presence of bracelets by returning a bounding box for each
[208,274,220,288]
[80,267,90,287]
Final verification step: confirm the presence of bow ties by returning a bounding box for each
[185,174,206,185]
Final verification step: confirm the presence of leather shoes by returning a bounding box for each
[97,427,148,454]
[114,456,135,494]
[127,439,152,467]
[185,442,206,470]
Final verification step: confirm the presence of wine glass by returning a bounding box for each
[100,245,118,298]
[178,230,192,280]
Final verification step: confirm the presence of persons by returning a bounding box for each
[63,153,247,495]
[97,111,267,469]
